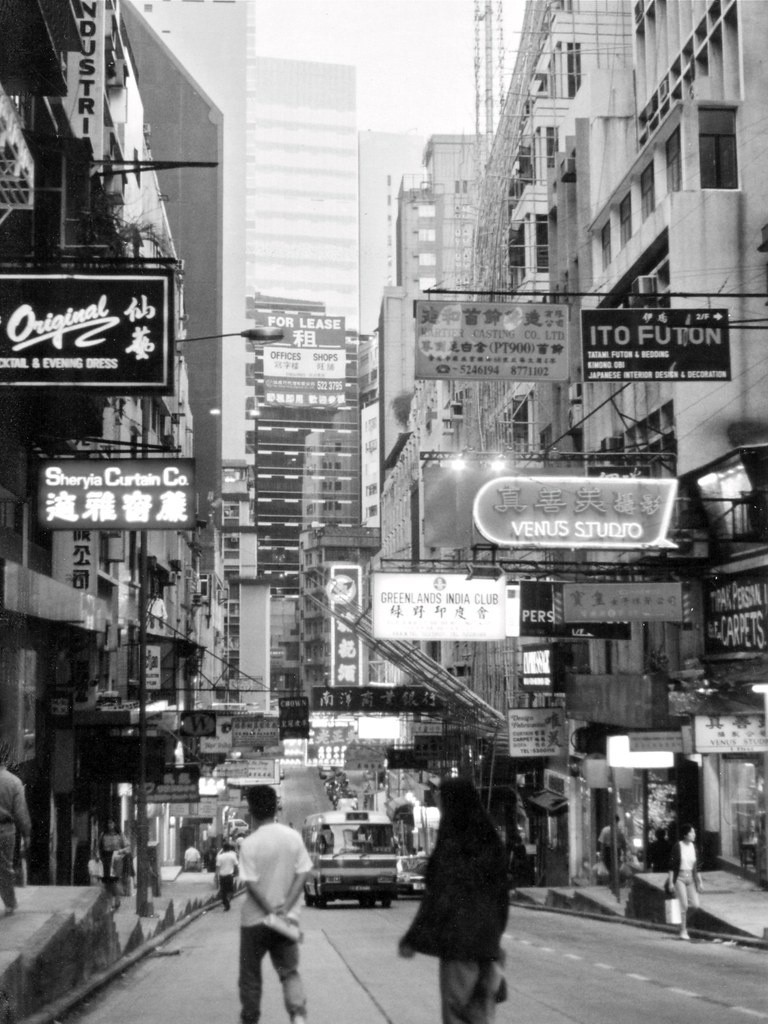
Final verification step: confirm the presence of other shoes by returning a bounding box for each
[3,900,19,916]
[113,901,121,909]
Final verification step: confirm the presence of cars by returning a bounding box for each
[394,855,430,896]
[227,818,249,837]
[318,767,333,779]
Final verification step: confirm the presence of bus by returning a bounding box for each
[302,809,402,908]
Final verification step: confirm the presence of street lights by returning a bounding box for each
[132,326,283,914]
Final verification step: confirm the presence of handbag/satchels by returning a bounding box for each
[88,858,104,878]
[490,960,507,1004]
[109,850,126,879]
[664,898,683,926]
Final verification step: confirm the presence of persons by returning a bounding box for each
[214,832,246,911]
[236,785,314,1024]
[96,814,132,912]
[182,841,203,873]
[0,744,33,922]
[666,825,704,941]
[597,815,627,896]
[646,827,670,872]
[399,779,511,1024]
